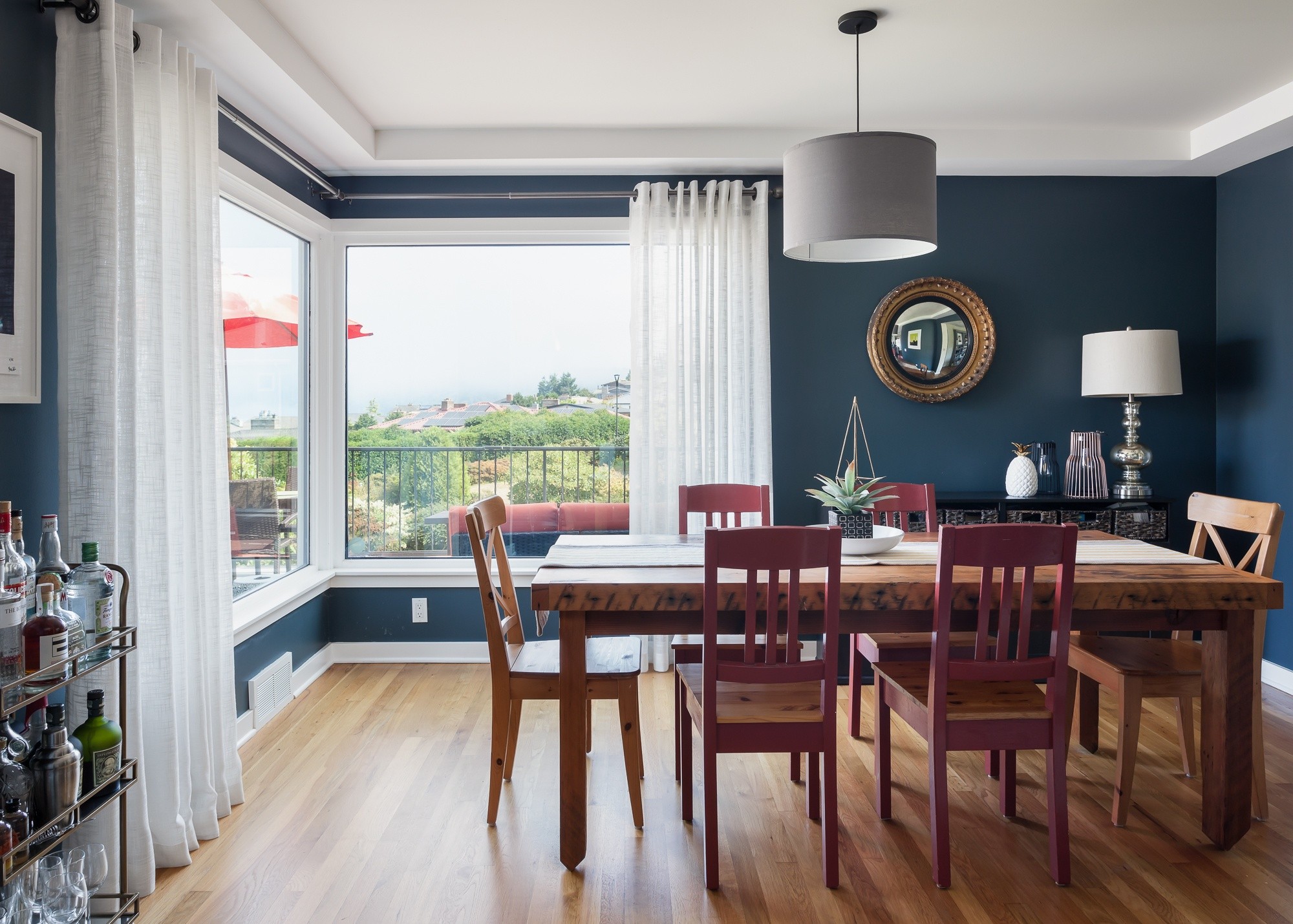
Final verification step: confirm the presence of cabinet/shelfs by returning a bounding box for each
[816,492,1176,687]
[0,562,140,924]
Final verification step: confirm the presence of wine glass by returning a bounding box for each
[0,842,108,924]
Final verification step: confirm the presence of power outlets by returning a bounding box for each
[412,598,428,623]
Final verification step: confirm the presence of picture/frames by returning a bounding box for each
[0,111,42,404]
[907,329,922,350]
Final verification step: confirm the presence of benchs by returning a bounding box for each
[450,502,630,558]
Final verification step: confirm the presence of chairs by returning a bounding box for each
[1069,489,1286,832]
[872,523,1077,892]
[226,467,298,583]
[465,491,647,834]
[661,483,806,785]
[672,528,843,892]
[825,480,942,740]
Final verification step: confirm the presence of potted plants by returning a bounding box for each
[801,460,900,538]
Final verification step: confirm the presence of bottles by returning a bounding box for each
[0,500,123,880]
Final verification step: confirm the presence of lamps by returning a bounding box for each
[782,9,935,264]
[1063,431,1108,499]
[1031,440,1061,495]
[1081,325,1182,495]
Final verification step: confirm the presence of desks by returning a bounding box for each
[425,511,451,557]
[276,491,298,574]
[530,536,1293,874]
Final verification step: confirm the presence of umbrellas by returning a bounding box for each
[222,270,374,481]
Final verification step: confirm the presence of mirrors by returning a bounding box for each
[867,275,997,405]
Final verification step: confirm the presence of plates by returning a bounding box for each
[804,524,905,556]
